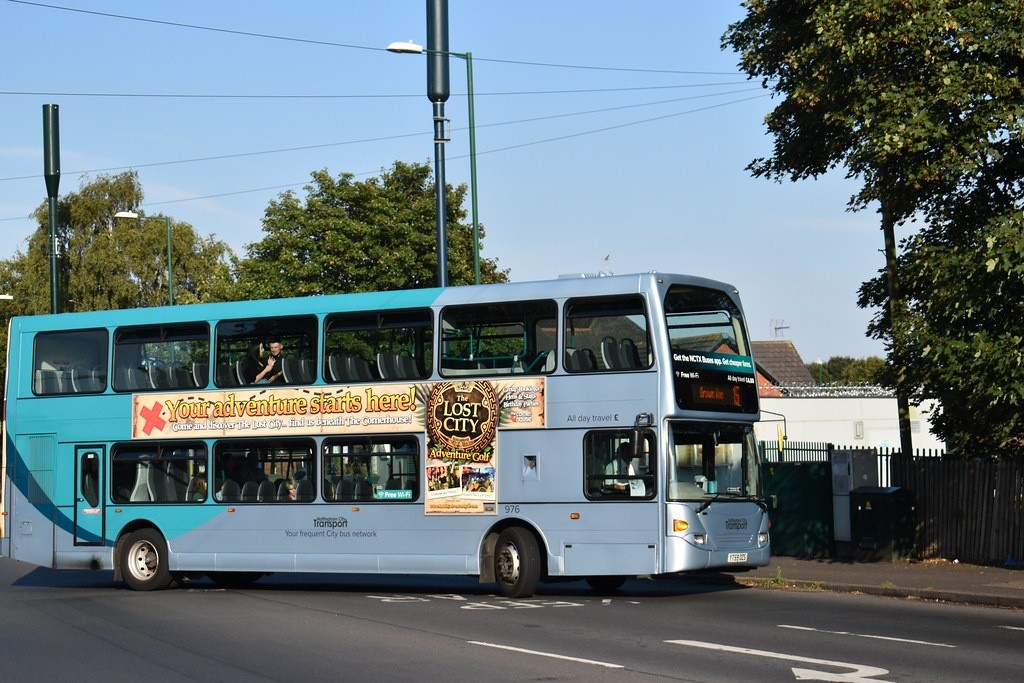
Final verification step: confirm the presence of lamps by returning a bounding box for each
[855,421,863,438]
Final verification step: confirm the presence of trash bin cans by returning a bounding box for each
[849,486,920,564]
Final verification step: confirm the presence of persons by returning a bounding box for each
[216,480,226,501]
[192,476,208,503]
[602,442,636,489]
[243,337,284,384]
[229,451,269,495]
[284,478,299,500]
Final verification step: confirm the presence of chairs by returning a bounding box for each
[32,336,642,394]
[84,447,418,503]
[586,455,610,497]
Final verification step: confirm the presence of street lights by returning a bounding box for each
[386,40,482,286]
[114,210,175,308]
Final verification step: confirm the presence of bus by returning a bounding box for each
[1,270,787,600]
